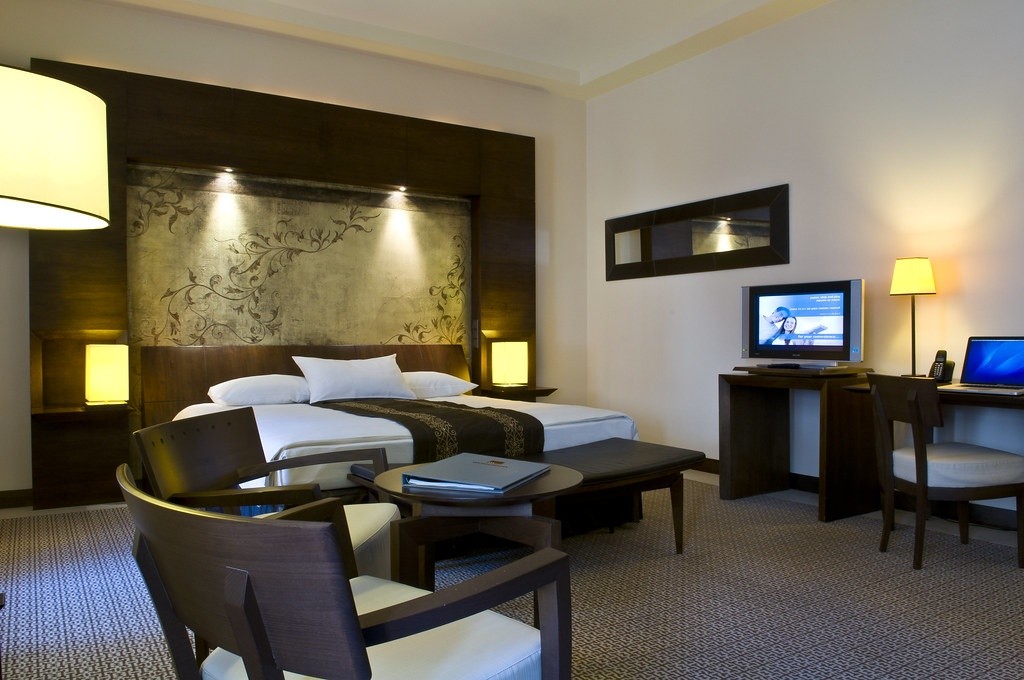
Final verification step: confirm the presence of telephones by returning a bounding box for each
[927,348,957,385]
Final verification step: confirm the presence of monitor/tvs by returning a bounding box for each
[741,278,865,369]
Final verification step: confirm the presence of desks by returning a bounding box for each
[715,373,896,523]
[540,437,707,554]
[843,375,1024,530]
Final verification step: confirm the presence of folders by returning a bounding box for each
[401,452,552,494]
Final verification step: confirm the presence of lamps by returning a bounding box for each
[0,63,109,613]
[889,256,937,379]
[84,344,129,409]
[490,341,529,391]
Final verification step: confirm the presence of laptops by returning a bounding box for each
[937,336,1024,395]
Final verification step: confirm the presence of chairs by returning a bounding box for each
[132,406,403,580]
[113,460,577,680]
[866,370,1024,570]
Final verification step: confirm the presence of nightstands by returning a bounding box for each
[30,408,130,511]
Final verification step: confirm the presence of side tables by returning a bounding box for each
[372,458,585,632]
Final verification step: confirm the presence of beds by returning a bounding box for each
[139,343,644,570]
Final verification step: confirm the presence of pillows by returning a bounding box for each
[401,371,480,400]
[290,354,418,405]
[206,374,311,406]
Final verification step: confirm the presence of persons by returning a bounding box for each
[759,306,815,346]
